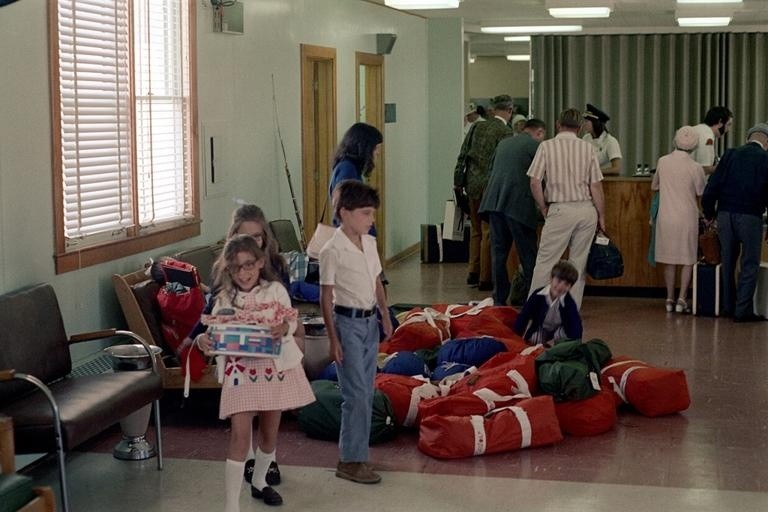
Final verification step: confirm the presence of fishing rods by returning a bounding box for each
[272,74,307,250]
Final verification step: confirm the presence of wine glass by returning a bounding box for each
[105,345,164,460]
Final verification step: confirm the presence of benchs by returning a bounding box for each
[111,219,335,389]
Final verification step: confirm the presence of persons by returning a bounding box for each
[454,93,622,314]
[325,122,389,301]
[195,234,317,511]
[647,107,768,324]
[319,179,393,487]
[513,259,583,346]
[182,205,293,484]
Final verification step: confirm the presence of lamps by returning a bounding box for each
[677,16,731,28]
[480,25,583,35]
[383,0,460,10]
[548,7,611,19]
[504,36,531,41]
[507,54,531,61]
[0,282,163,512]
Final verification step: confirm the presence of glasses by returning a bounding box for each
[251,232,265,241]
[229,258,258,274]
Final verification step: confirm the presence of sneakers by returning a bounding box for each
[335,459,382,484]
[632,164,650,176]
[733,314,760,323]
[467,272,493,291]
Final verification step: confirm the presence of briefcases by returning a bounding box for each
[692,256,724,317]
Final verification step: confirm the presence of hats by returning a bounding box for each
[747,123,768,140]
[511,114,527,130]
[465,102,477,116]
[672,125,698,151]
[581,103,609,123]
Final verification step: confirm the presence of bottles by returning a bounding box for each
[635,164,649,175]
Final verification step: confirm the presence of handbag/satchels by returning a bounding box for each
[699,225,721,264]
[304,223,339,285]
[420,199,470,264]
[586,222,624,280]
[290,296,529,460]
[144,256,210,352]
[530,339,690,445]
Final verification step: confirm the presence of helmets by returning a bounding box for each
[665,297,691,313]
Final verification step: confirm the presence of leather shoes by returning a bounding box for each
[265,460,281,485]
[251,485,282,505]
[244,459,256,483]
